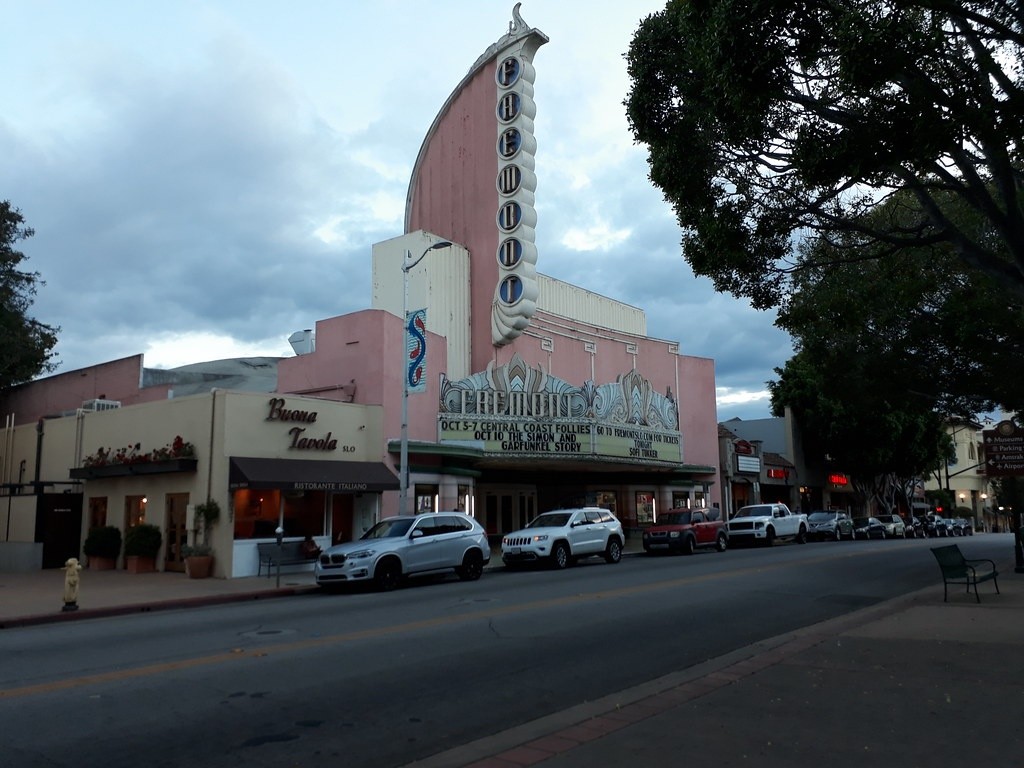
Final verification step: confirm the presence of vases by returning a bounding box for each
[69,464,133,479]
[133,460,197,474]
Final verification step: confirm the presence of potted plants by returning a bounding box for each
[181,500,219,579]
[124,525,163,574]
[83,526,121,571]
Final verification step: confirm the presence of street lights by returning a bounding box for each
[397,242,453,518]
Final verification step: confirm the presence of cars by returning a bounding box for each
[313,512,491,593]
[902,515,974,538]
[852,516,887,540]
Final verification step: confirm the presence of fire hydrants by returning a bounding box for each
[61,558,82,611]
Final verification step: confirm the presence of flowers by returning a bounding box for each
[132,435,195,462]
[82,443,141,468]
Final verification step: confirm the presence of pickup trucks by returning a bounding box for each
[642,505,729,556]
[724,503,811,547]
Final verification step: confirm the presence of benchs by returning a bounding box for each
[930,544,1000,603]
[257,540,322,578]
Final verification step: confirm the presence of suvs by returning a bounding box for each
[500,507,626,570]
[808,509,855,541]
[873,514,906,539]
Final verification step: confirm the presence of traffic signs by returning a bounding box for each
[982,420,1024,478]
